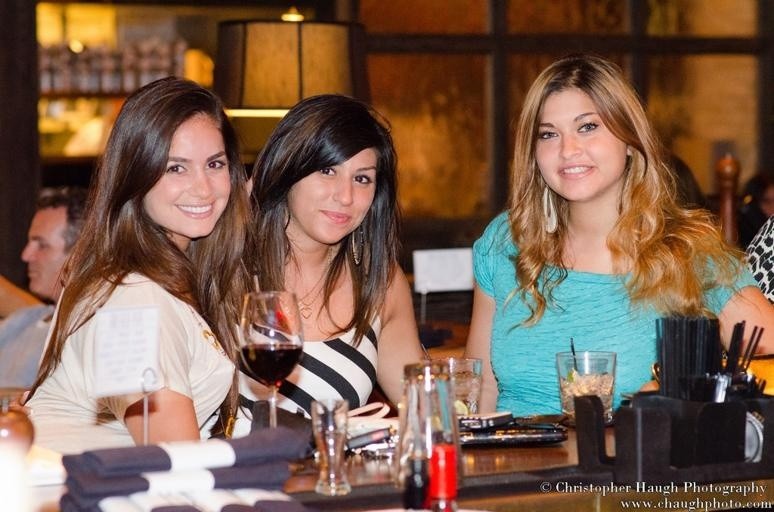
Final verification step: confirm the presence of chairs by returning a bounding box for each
[413,246,478,341]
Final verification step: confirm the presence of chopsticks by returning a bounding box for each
[655,315,765,402]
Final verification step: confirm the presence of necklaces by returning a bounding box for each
[294,246,333,319]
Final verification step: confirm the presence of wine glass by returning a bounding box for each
[237,289,305,429]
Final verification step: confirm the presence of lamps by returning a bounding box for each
[218,20,356,117]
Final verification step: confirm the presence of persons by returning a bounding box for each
[1,186,91,388]
[738,212,773,306]
[666,150,704,210]
[1,273,45,318]
[736,177,773,250]
[20,75,238,456]
[198,92,429,441]
[458,49,773,418]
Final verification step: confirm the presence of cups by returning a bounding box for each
[447,357,482,416]
[555,349,617,423]
[311,399,352,497]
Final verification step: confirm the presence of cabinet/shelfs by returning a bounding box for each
[35,1,220,202]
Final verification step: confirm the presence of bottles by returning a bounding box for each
[38,38,172,93]
[397,359,465,511]
